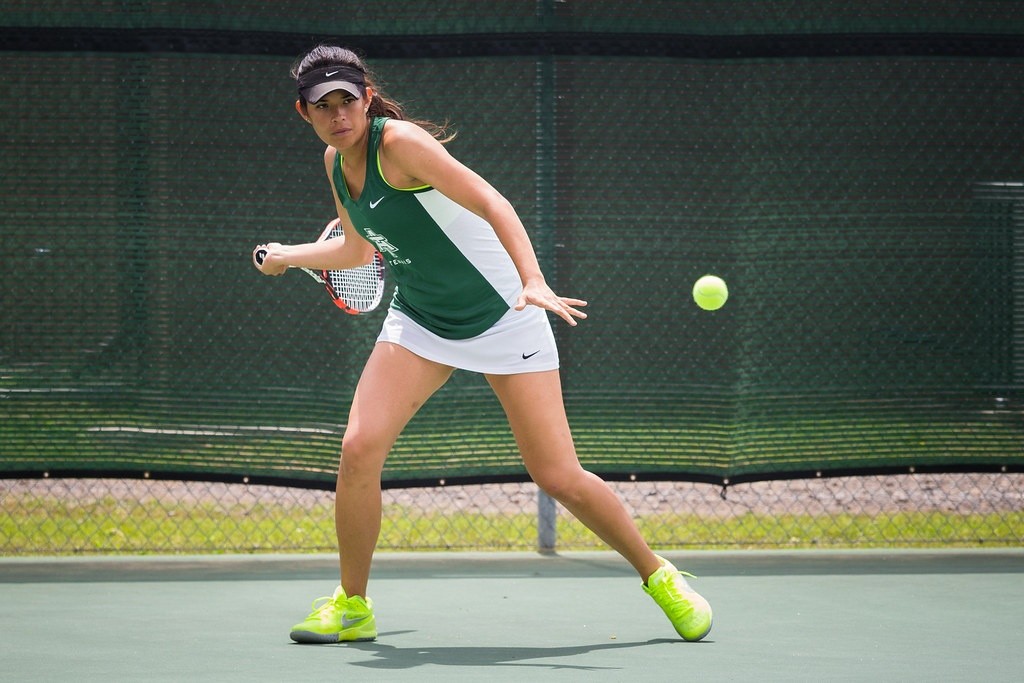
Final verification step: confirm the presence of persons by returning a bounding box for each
[254,46,712,646]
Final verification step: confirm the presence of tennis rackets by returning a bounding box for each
[253,217,386,316]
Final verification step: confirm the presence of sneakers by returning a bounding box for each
[290,585,377,642]
[641,554,712,641]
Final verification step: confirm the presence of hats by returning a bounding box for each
[298,66,365,105]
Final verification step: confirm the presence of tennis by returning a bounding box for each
[692,275,729,311]
